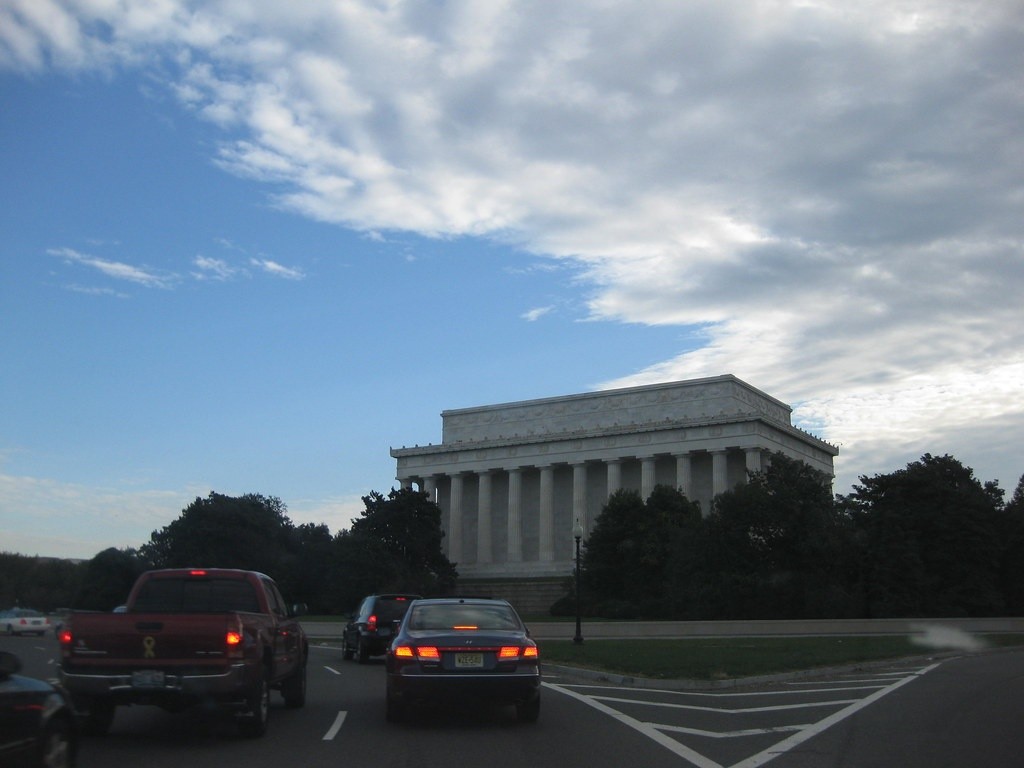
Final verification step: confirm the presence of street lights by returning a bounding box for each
[572,518,586,647]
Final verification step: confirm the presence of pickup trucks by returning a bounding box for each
[58,566,309,737]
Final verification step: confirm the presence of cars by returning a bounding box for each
[0,606,50,636]
[0,652,74,768]
[386,596,541,726]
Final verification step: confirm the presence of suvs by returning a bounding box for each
[344,589,407,665]
